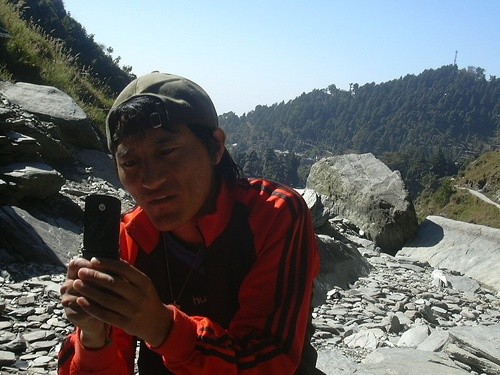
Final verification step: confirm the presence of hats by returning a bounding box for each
[105,70,219,154]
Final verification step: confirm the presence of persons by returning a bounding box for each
[58,71,326,375]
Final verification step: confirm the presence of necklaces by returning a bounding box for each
[162,231,203,309]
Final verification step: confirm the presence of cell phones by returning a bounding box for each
[81,195,121,280]
